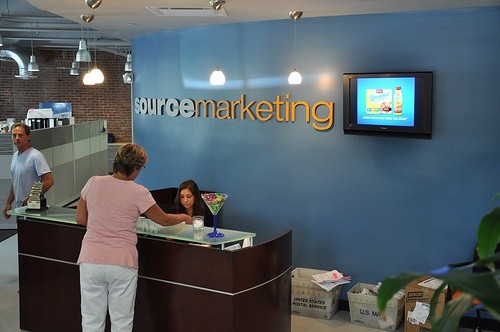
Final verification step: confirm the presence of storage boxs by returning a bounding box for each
[291,267,343,320]
[405,275,450,332]
[347,282,406,332]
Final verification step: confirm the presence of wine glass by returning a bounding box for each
[202,193,228,240]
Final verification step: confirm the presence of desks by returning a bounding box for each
[6,204,292,332]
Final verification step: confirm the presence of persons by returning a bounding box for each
[170,180,213,227]
[3,123,54,293]
[76,143,193,332]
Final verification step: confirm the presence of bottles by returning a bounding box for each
[394,86,402,115]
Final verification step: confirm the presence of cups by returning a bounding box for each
[6,118,16,133]
[193,215,204,240]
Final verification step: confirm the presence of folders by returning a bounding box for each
[43,119,46,128]
[32,119,35,129]
[45,118,49,128]
[28,119,32,129]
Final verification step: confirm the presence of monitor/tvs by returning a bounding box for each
[343,71,433,139]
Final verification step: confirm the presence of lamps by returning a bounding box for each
[288,11,303,85]
[70,0,104,85]
[123,42,133,84]
[209,0,225,85]
[28,40,39,71]
